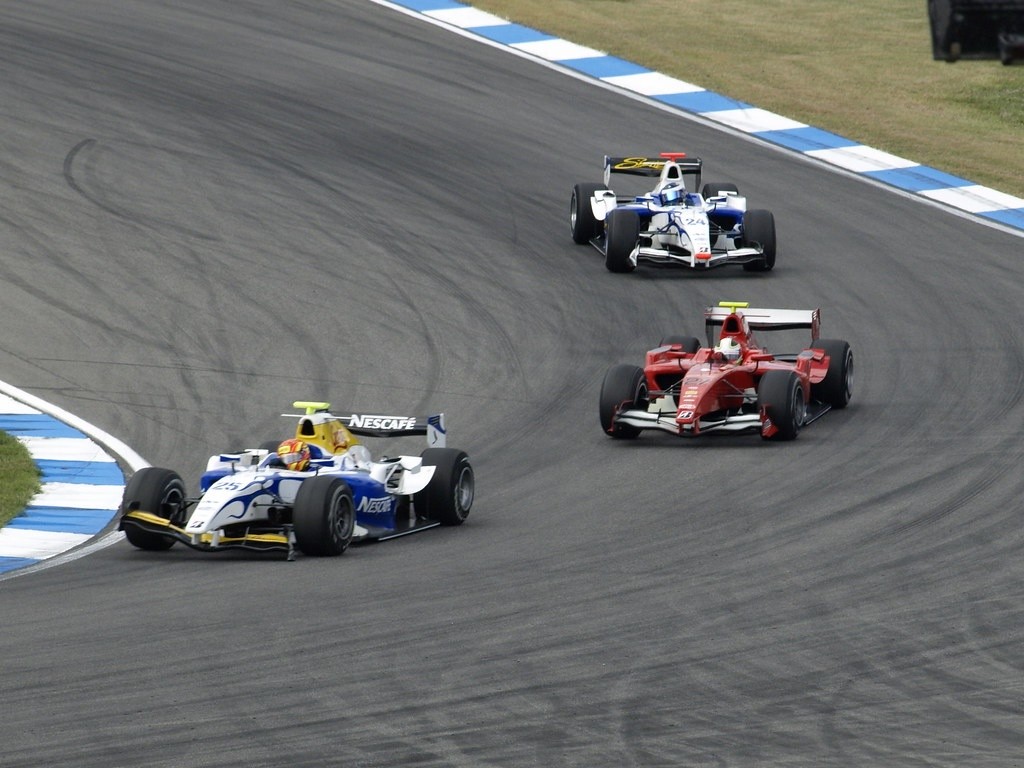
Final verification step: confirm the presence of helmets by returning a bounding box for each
[714,337,743,365]
[661,181,683,204]
[277,439,310,471]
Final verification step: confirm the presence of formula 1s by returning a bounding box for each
[598,302,855,443]
[568,151,777,273]
[115,402,476,562]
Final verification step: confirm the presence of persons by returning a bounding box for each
[713,337,742,364]
[660,182,694,206]
[277,438,315,472]
